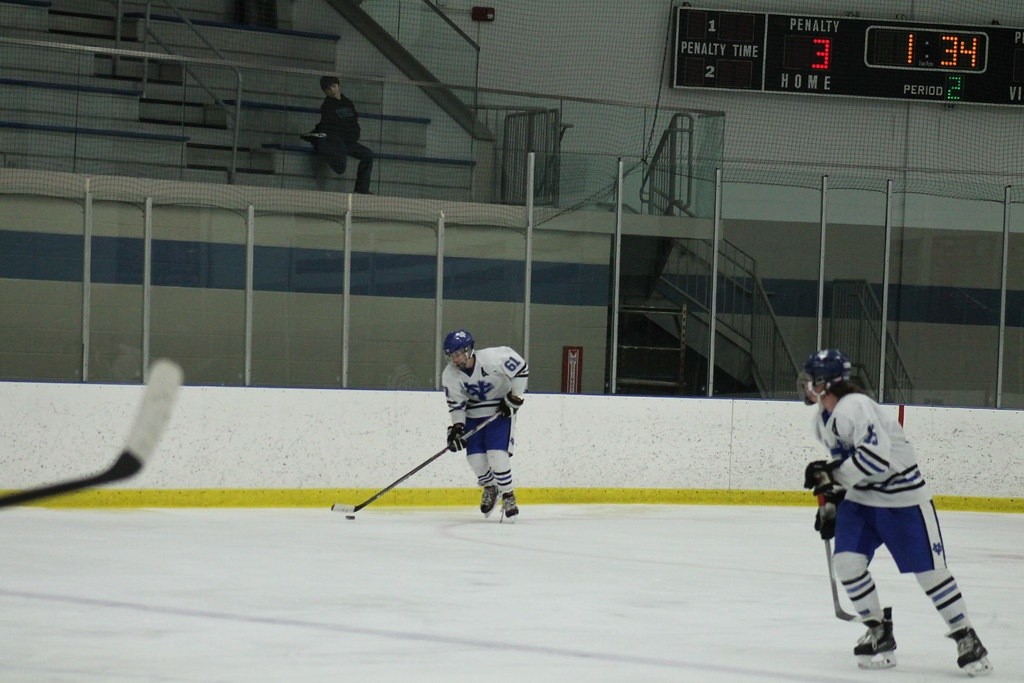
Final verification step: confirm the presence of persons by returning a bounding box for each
[442,330,529,524]
[796,348,994,677]
[300,76,375,194]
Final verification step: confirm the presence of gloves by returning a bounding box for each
[495,390,524,420]
[814,498,841,541]
[447,423,469,452]
[804,458,842,496]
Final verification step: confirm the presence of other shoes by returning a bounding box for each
[299,132,327,143]
[354,188,374,195]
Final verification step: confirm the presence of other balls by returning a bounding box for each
[345,515,355,520]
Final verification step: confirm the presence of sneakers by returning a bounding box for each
[500,490,519,523]
[945,626,994,678]
[480,485,499,518]
[854,607,897,670]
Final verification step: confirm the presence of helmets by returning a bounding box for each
[796,348,853,406]
[444,329,475,369]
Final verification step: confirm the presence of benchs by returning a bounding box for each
[0,0,477,203]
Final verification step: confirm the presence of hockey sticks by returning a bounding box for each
[813,463,859,624]
[330,410,503,514]
[0,356,187,513]
